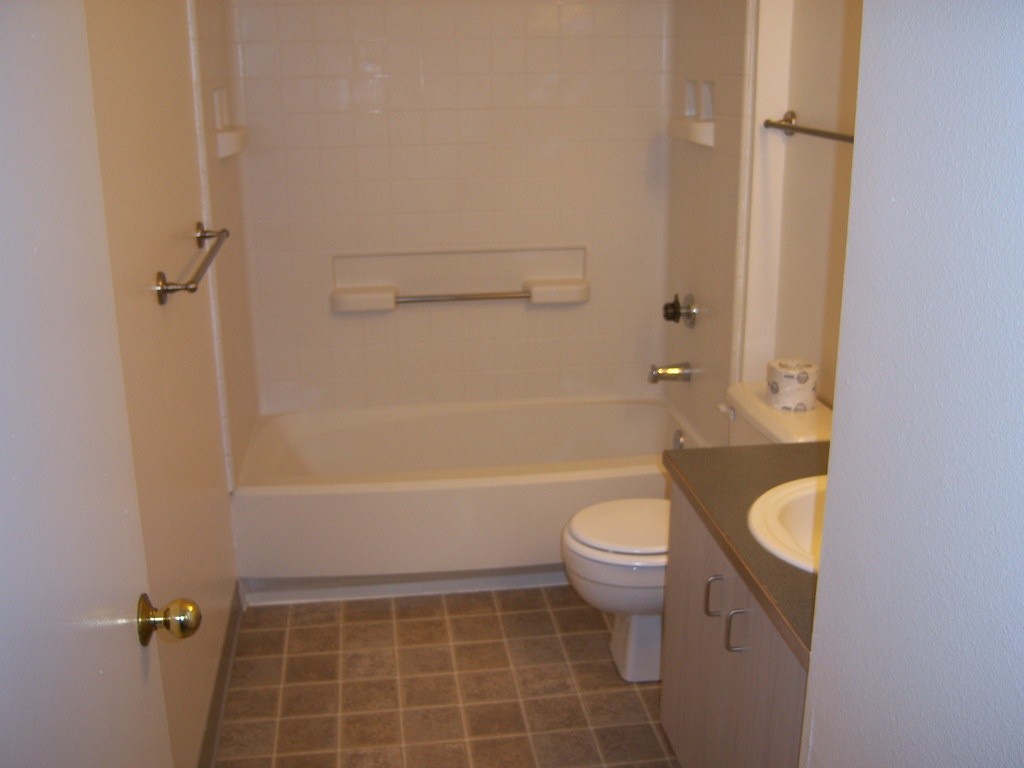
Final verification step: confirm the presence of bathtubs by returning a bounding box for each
[234,396,719,607]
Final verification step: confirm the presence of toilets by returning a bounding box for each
[561,381,833,682]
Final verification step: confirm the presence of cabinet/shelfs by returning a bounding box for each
[658,440,830,768]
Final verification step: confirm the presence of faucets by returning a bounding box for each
[646,362,692,384]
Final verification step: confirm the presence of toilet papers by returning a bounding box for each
[764,358,820,412]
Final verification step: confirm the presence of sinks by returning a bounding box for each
[745,475,828,575]
